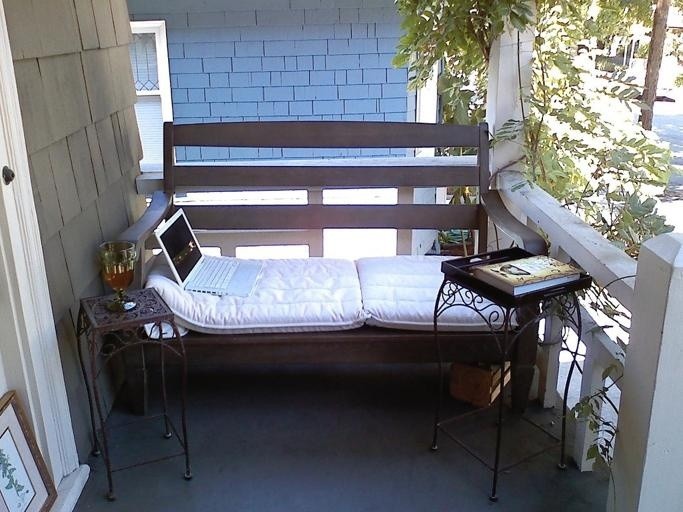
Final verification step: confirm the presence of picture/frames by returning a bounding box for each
[0,390,58,512]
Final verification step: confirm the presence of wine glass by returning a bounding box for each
[97,241,137,313]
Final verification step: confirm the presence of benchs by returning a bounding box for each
[116,121,548,416]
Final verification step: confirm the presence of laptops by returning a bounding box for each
[155,208,265,297]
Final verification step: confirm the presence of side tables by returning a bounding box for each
[430,273,581,502]
[77,287,192,500]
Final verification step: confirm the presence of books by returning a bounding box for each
[471,254,581,297]
